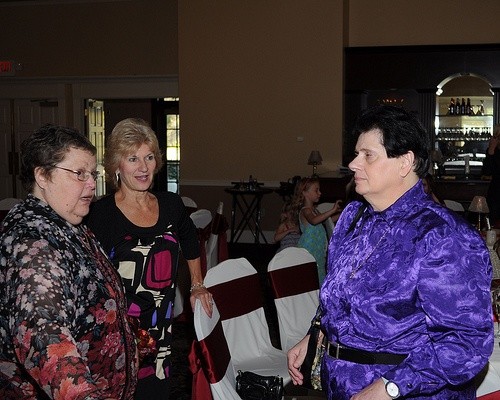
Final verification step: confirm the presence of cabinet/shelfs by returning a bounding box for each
[433,114,494,142]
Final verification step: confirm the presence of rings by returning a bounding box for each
[209,301,214,304]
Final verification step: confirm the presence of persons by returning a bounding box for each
[287,104,494,400]
[277,178,342,287]
[81,118,214,400]
[422,126,500,225]
[0,127,139,400]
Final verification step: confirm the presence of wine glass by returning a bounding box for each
[438,127,491,141]
[492,291,500,339]
[480,100,485,115]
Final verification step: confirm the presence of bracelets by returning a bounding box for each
[189,282,204,292]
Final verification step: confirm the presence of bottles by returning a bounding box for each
[433,162,439,180]
[466,98,471,115]
[461,98,466,114]
[248,176,253,191]
[448,98,455,115]
[464,156,471,180]
[455,98,461,115]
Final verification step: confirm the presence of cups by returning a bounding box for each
[253,178,257,184]
[486,230,497,246]
[470,105,481,115]
[250,185,256,192]
[239,184,248,192]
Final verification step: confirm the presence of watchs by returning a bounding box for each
[381,376,401,399]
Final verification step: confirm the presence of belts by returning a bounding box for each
[325,341,408,365]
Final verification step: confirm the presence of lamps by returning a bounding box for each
[309,150,323,179]
[468,196,490,233]
[0,59,25,78]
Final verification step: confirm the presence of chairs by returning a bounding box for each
[176,195,321,400]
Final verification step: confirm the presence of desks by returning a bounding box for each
[225,186,276,248]
[278,179,492,222]
[475,322,500,400]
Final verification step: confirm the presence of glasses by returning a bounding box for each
[48,164,100,181]
